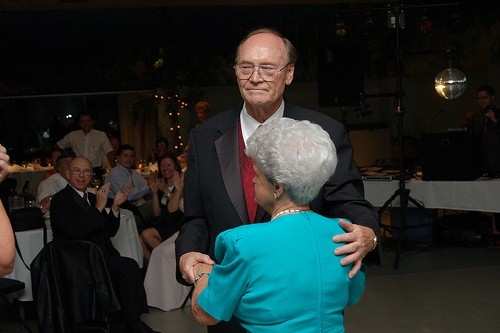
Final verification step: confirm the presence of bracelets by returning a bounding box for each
[194,272,209,286]
[166,191,171,196]
[371,235,378,251]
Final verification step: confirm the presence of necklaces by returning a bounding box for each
[271,209,298,220]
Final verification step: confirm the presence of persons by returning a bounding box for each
[462,85,500,177]
[190,116,368,333]
[0,101,212,333]
[174,28,378,333]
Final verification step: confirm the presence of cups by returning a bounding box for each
[486,104,494,115]
[415,166,423,181]
[26,200,38,207]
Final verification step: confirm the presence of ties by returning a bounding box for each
[83,192,87,203]
[241,125,262,224]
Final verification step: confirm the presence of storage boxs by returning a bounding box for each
[389,207,438,241]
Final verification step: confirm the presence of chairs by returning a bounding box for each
[30,239,158,333]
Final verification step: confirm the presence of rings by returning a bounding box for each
[102,189,106,193]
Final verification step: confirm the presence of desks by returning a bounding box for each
[4,165,55,212]
[0,208,145,320]
[363,175,500,254]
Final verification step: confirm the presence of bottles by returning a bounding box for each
[135,159,159,173]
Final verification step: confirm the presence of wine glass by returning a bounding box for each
[9,157,53,170]
[92,178,100,193]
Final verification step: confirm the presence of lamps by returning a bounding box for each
[415,6,433,35]
[333,12,350,41]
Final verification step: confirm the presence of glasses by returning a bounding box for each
[233,60,291,82]
[70,168,92,176]
